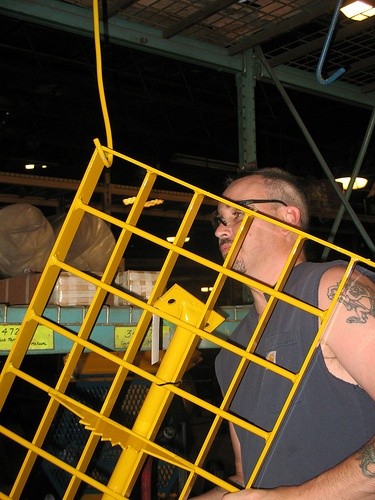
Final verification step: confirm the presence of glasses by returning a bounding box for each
[210,200,287,230]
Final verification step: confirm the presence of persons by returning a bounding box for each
[186,167,375,500]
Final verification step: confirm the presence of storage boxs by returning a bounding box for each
[0,267,166,307]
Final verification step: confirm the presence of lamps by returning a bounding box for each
[339,0,375,22]
